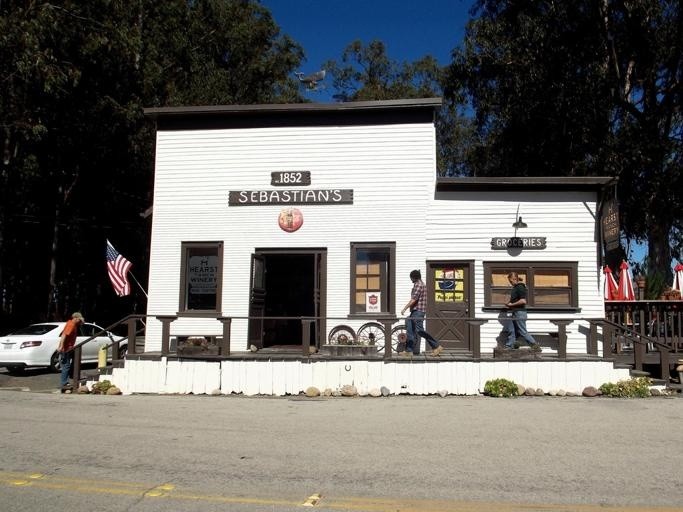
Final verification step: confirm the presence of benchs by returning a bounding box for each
[169,334,223,345]
[323,344,378,356]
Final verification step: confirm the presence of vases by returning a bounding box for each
[177,346,220,356]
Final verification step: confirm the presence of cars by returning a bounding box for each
[0,320,129,377]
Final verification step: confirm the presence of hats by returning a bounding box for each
[72,312,82,317]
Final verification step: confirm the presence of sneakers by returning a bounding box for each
[432,345,443,357]
[399,351,413,356]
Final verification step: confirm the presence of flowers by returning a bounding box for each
[186,338,209,346]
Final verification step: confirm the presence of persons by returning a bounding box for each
[399,269,444,357]
[500,272,542,351]
[56,311,84,393]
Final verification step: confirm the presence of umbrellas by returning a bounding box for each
[602,263,618,300]
[616,259,636,324]
[672,261,682,298]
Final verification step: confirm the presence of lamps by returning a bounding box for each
[512,203,528,237]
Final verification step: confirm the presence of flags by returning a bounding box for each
[105,239,133,297]
